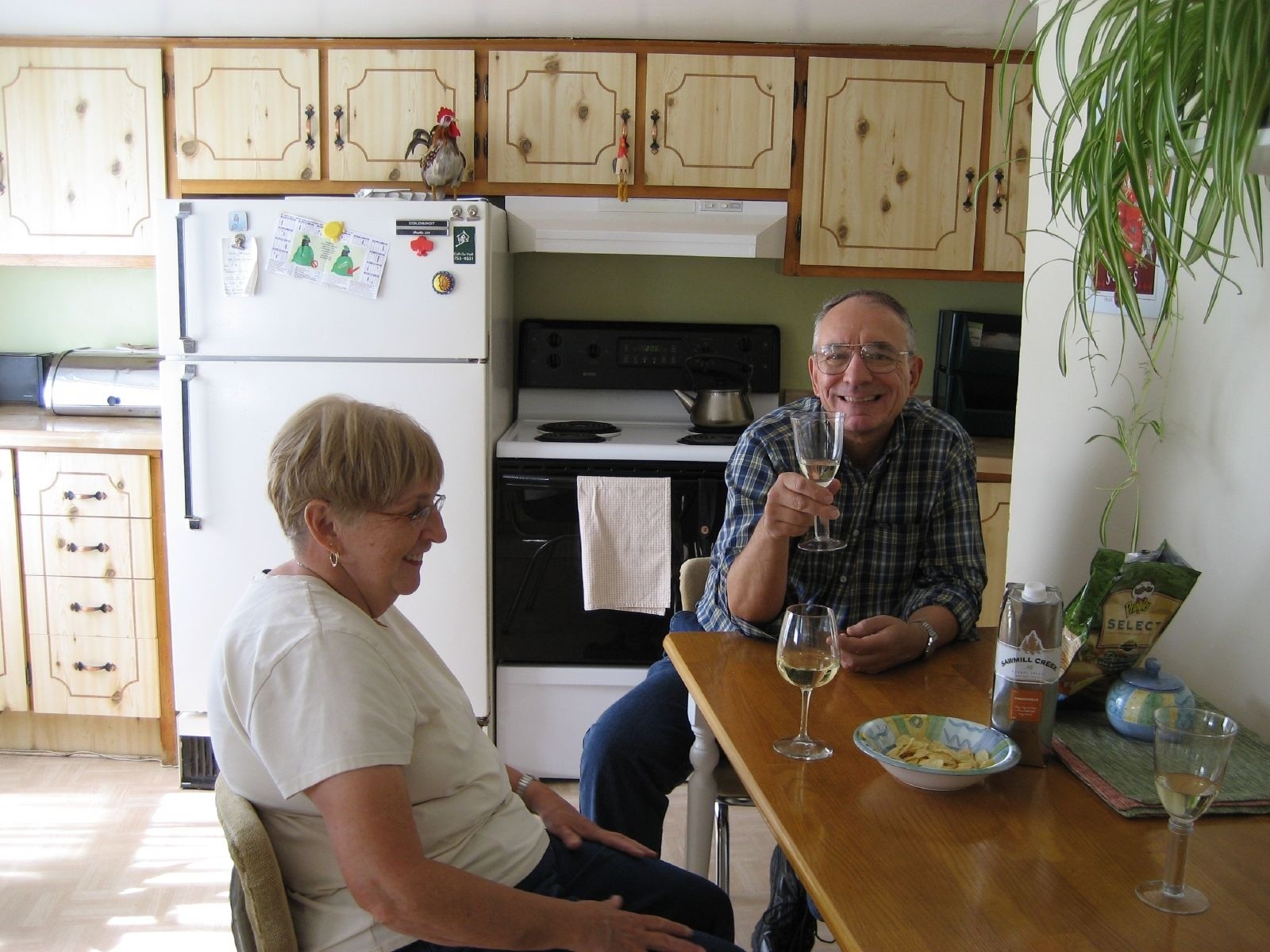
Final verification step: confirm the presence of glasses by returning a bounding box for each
[361,494,446,530]
[813,341,911,376]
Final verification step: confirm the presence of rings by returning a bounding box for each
[854,667,861,672]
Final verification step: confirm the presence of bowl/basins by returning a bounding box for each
[1104,656,1197,743]
[852,713,1023,792]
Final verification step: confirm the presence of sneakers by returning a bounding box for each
[750,897,838,952]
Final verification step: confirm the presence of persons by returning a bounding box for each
[208,393,748,952]
[578,289,988,952]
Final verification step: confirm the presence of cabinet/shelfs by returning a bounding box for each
[785,43,1036,281]
[0,35,170,268]
[973,480,1011,628]
[0,447,180,766]
[170,39,482,196]
[482,39,800,201]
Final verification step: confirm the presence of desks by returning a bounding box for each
[663,629,1269,952]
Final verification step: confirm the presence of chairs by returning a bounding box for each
[214,769,297,952]
[678,557,756,896]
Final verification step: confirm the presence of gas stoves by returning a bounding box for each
[495,317,782,462]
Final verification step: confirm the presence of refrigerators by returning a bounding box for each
[156,198,516,793]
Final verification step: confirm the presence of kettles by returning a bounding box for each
[672,353,756,430]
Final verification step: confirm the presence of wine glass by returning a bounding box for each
[789,411,847,552]
[772,604,841,761]
[1132,705,1238,915]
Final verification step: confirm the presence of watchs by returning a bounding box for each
[909,621,939,660]
[515,772,540,798]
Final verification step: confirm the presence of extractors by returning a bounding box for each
[505,196,788,259]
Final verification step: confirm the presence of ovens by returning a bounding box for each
[492,457,728,667]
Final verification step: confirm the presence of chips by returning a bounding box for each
[887,734,995,770]
